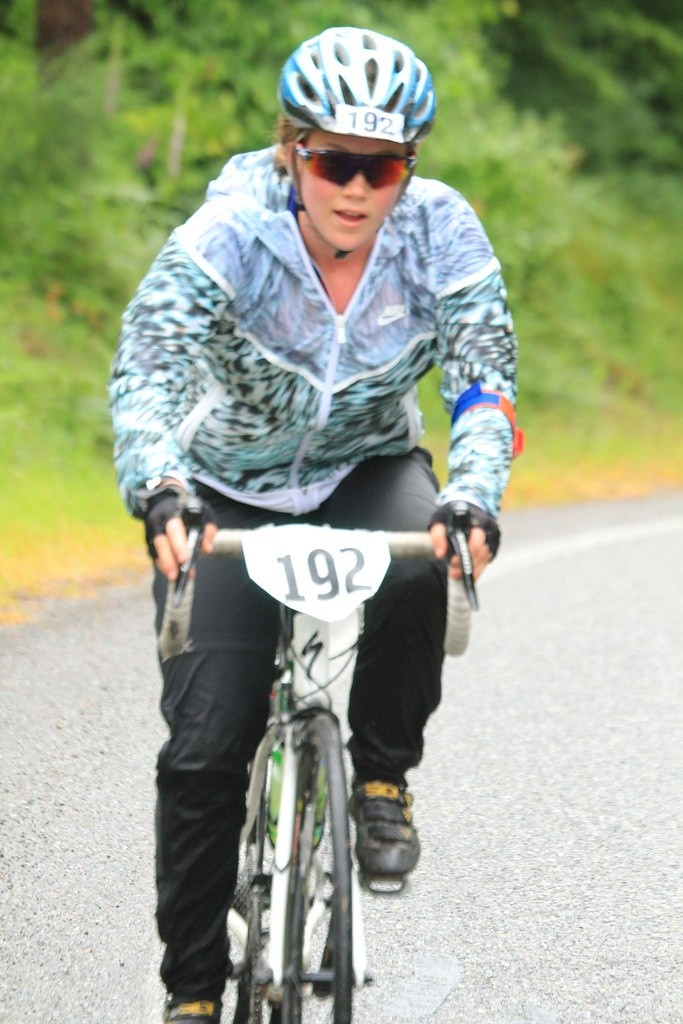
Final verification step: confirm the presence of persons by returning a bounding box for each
[105,26,522,1024]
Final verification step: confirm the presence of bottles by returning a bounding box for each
[268,741,326,855]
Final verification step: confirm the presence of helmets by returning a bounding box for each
[277,27,433,142]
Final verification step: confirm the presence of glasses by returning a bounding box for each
[292,140,418,189]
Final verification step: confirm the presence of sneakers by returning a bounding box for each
[166,997,222,1024]
[349,780,420,876]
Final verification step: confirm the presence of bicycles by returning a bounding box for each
[153,496,482,1024]
